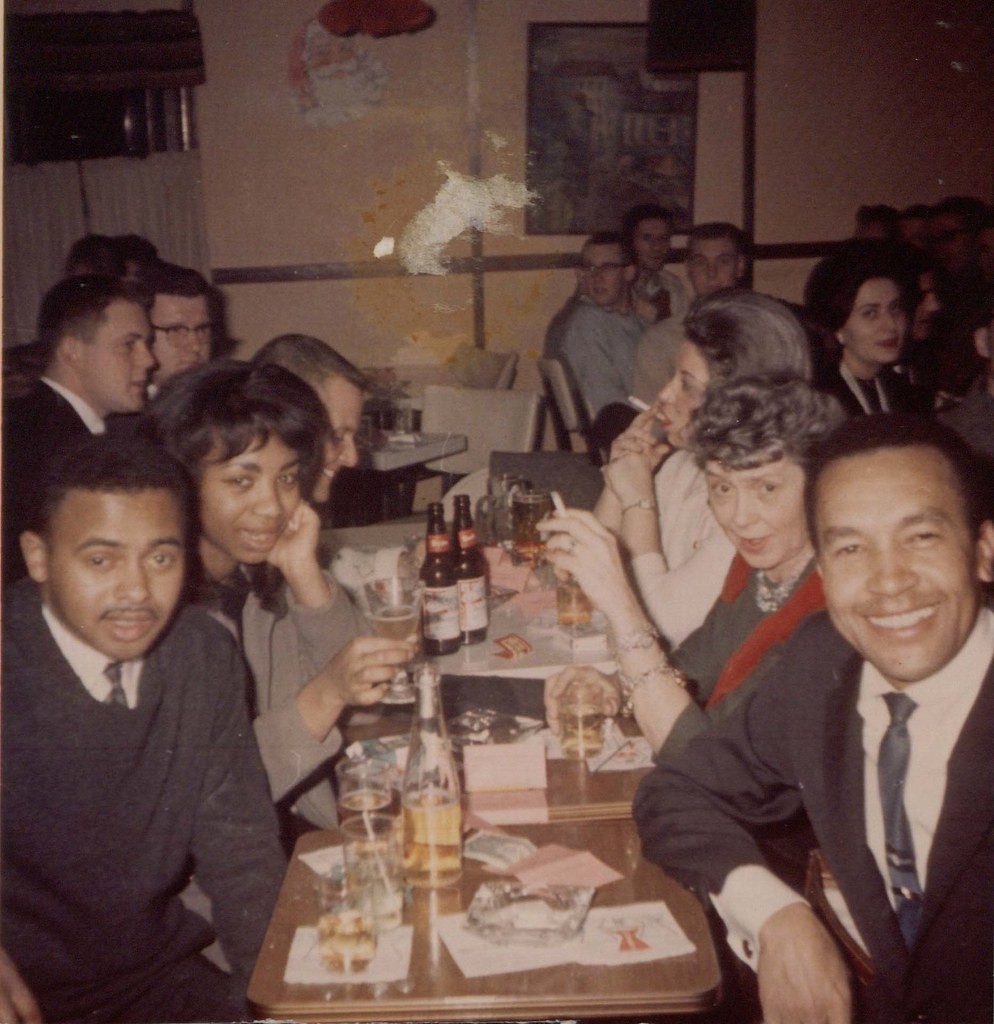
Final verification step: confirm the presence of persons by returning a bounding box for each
[631,409,993,1023]
[0,428,292,1023]
[2,232,414,833]
[536,194,994,884]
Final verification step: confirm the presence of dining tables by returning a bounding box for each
[245,364,724,1024]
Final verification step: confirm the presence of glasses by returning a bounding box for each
[150,321,218,347]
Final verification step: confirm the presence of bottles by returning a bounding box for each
[451,494,490,646]
[417,502,462,656]
[401,662,463,889]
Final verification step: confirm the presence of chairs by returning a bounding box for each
[419,384,539,498]
[448,339,520,390]
[485,448,608,540]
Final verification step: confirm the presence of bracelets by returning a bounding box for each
[619,662,688,720]
[613,623,660,659]
[622,501,660,518]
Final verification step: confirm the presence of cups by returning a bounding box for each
[336,759,395,826]
[317,867,378,975]
[476,473,532,549]
[560,683,604,760]
[514,489,555,567]
[340,814,407,931]
[556,579,593,629]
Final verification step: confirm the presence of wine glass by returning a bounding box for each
[363,577,425,704]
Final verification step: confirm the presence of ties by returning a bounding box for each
[877,693,923,953]
[103,662,130,709]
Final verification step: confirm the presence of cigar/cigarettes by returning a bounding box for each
[550,490,567,518]
[627,395,665,423]
